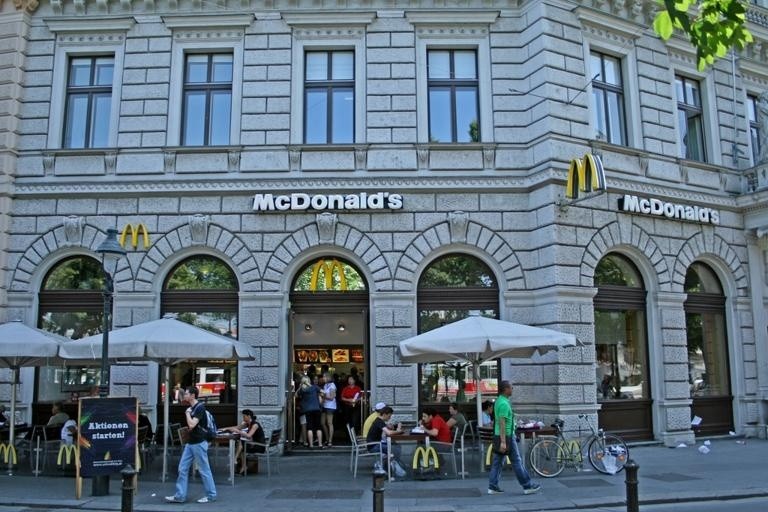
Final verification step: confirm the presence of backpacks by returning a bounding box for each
[191,403,216,438]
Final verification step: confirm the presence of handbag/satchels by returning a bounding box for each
[491,436,512,455]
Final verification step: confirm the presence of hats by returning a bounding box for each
[375,403,385,410]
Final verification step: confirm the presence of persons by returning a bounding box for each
[138,406,152,447]
[60,414,78,446]
[445,403,469,450]
[422,375,437,402]
[0,405,7,422]
[294,364,363,450]
[479,400,505,468]
[456,380,467,402]
[486,380,541,495]
[45,401,71,426]
[419,406,453,473]
[163,386,218,503]
[364,401,396,475]
[366,406,404,480]
[219,408,266,475]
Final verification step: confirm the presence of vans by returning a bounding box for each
[162,363,237,406]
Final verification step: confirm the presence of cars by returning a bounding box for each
[610,376,643,400]
[40,368,107,404]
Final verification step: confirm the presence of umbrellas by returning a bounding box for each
[395,311,576,463]
[0,317,74,471]
[62,313,258,480]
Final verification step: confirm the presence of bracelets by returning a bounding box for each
[240,431,246,437]
[238,424,244,429]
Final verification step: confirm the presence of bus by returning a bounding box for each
[419,358,503,403]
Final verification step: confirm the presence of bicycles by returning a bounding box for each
[529,412,630,478]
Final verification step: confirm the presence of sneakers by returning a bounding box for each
[523,484,540,495]
[165,496,183,503]
[487,487,504,495]
[197,497,215,503]
